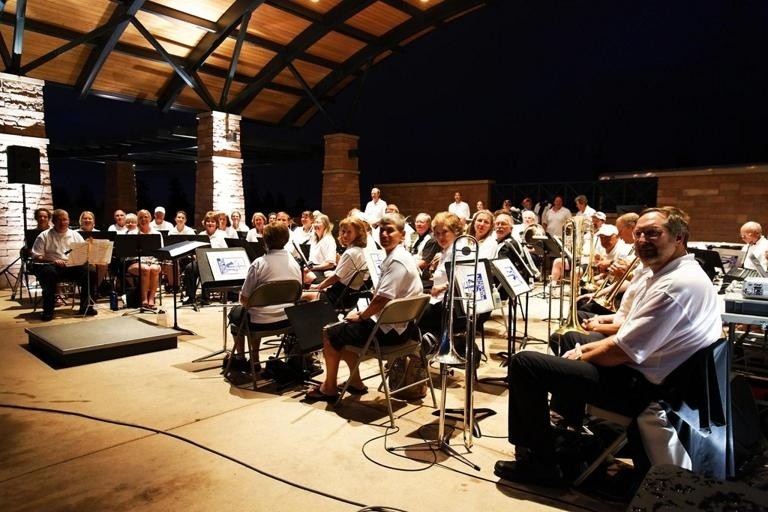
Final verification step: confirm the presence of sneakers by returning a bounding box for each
[78,307,98,315]
[41,313,53,321]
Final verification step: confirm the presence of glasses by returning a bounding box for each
[632,229,664,240]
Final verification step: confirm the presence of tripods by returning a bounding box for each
[475,296,548,386]
[432,311,497,439]
[0,255,233,365]
[530,244,560,299]
[273,347,344,393]
[386,286,483,471]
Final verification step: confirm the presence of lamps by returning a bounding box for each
[348,149,359,160]
[223,132,238,143]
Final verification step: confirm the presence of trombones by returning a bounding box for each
[434,235,480,448]
[588,247,641,312]
[555,219,589,356]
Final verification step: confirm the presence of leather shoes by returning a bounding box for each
[494,459,561,488]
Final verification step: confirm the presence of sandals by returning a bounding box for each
[304,385,338,404]
[338,380,367,394]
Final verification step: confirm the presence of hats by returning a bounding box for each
[597,224,620,238]
[154,206,167,215]
[591,210,608,222]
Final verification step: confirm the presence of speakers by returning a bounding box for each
[7,146,41,185]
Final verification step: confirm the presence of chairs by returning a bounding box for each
[225,257,533,429]
[19,240,226,315]
[520,239,741,487]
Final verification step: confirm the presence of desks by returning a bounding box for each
[627,464,768,512]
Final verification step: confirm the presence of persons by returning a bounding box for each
[25,209,63,307]
[72,211,107,299]
[32,209,97,321]
[735,221,768,330]
